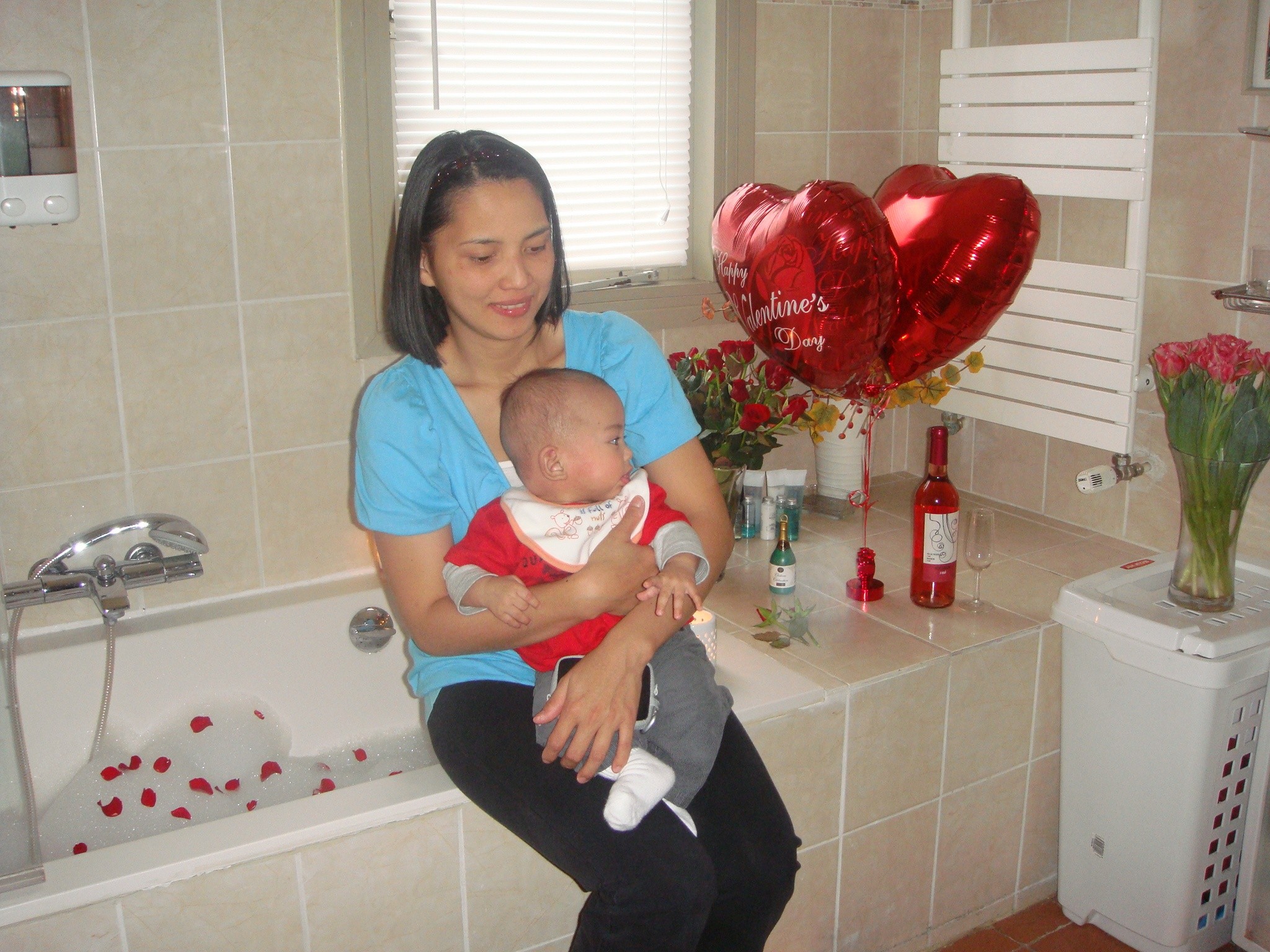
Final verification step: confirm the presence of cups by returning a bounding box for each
[1244,245,1270,310]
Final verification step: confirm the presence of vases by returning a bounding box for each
[1168,443,1270,612]
[713,464,748,527]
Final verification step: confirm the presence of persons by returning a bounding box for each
[350,129,804,952]
[442,369,735,837]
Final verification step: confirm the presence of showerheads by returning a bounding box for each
[62,512,208,559]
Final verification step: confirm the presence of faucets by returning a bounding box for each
[84,552,131,625]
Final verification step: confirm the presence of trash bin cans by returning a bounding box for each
[1050,547,1270,952]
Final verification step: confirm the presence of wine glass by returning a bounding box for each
[957,508,995,614]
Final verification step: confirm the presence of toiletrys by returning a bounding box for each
[737,467,811,542]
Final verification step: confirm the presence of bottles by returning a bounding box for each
[768,514,797,595]
[909,425,960,608]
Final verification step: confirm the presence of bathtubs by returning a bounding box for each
[5,564,826,949]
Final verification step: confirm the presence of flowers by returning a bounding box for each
[1147,331,1270,599]
[753,596,820,647]
[667,341,812,517]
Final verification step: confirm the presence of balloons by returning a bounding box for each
[873,164,1043,392]
[709,180,901,401]
